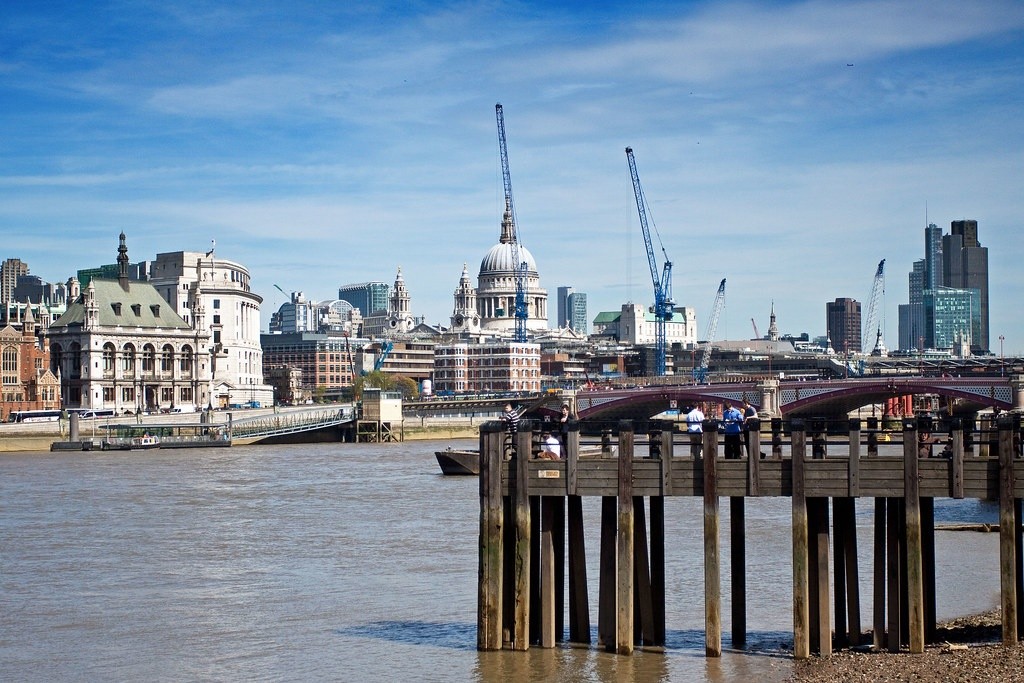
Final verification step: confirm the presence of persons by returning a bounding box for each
[539,430,564,460]
[721,401,747,459]
[498,403,520,454]
[686,403,708,459]
[558,403,579,458]
[742,398,767,458]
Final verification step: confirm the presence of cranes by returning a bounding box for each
[856,259,887,377]
[494,102,527,344]
[692,279,728,386]
[625,146,676,376]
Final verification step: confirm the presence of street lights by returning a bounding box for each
[999,335,1005,377]
[843,339,849,378]
[919,336,925,377]
[767,345,772,380]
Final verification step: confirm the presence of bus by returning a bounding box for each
[61,408,91,419]
[8,409,61,423]
[79,410,114,419]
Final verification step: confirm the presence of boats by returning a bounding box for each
[98,424,233,448]
[434,446,481,476]
[131,435,161,450]
